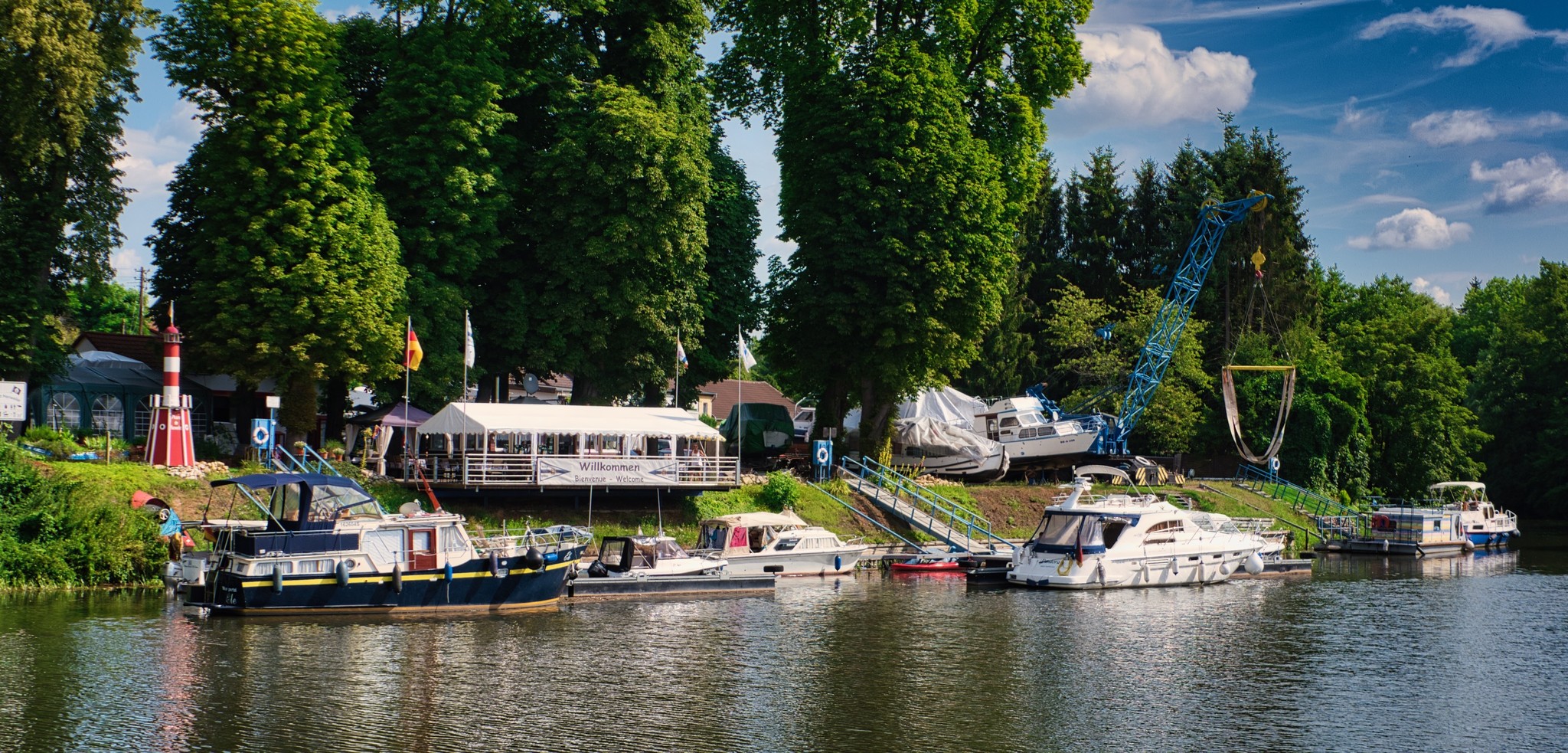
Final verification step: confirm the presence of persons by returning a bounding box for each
[1032,532,1048,552]
[261,448,277,467]
[686,443,705,483]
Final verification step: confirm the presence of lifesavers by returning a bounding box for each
[817,448,828,463]
[252,427,269,444]
[1377,515,1389,527]
[1272,458,1280,470]
[1135,456,1150,466]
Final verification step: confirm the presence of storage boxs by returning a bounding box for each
[399,469,403,477]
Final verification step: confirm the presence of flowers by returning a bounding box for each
[294,440,308,448]
[332,447,346,454]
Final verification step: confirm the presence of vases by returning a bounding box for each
[296,448,304,454]
[335,454,343,460]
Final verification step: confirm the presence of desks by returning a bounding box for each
[387,462,402,469]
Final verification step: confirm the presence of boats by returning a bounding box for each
[788,378,1104,484]
[1005,463,1284,588]
[126,470,594,615]
[567,487,729,576]
[1347,479,1517,555]
[688,509,869,575]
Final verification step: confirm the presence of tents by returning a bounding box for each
[343,401,727,486]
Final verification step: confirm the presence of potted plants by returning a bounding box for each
[361,428,374,458]
[318,448,329,458]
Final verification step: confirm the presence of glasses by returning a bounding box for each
[694,446,698,447]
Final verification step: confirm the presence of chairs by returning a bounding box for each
[492,458,507,480]
[399,454,413,479]
[417,458,433,479]
[449,459,458,479]
[393,455,403,476]
[469,460,474,479]
[473,461,493,479]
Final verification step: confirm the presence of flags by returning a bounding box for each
[465,310,475,369]
[1075,526,1083,568]
[677,329,688,369]
[403,316,423,371]
[739,324,757,373]
